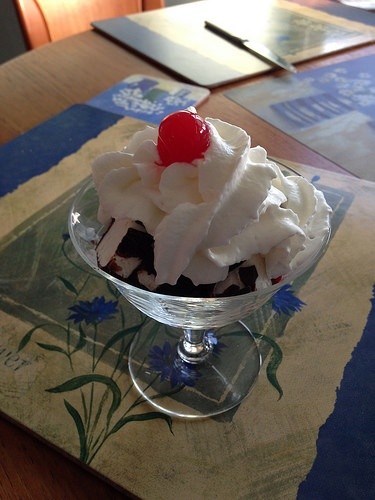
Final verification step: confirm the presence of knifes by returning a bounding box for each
[205,20,298,74]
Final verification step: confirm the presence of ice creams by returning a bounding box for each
[90,107,334,303]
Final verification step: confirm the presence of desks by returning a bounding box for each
[0,1,373,499]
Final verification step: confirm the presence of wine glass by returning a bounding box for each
[67,156,331,419]
